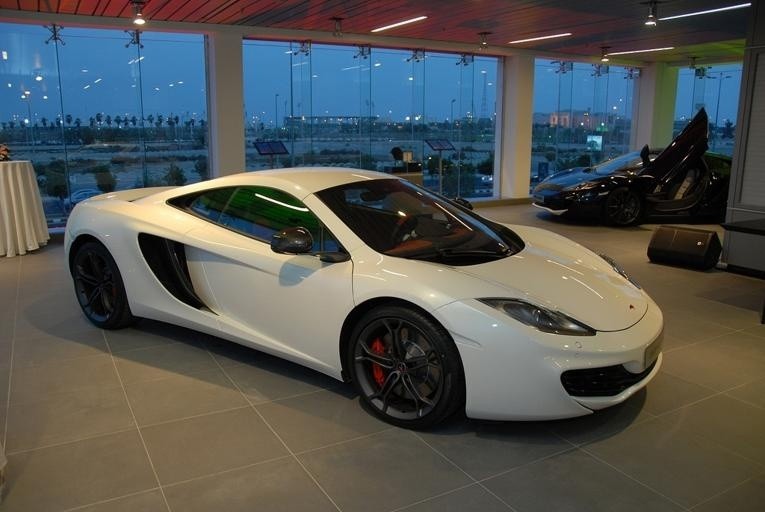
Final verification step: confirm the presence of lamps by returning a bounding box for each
[601,49,609,62]
[133,0,146,26]
[478,34,489,50]
[644,4,658,26]
[331,17,344,37]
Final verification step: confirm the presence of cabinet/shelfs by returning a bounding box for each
[720,220,765,280]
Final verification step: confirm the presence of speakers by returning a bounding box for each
[646,223,722,272]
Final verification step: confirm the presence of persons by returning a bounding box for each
[389,146,404,167]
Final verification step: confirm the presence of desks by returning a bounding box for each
[1,159,51,258]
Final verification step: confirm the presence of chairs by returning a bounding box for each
[672,169,704,201]
